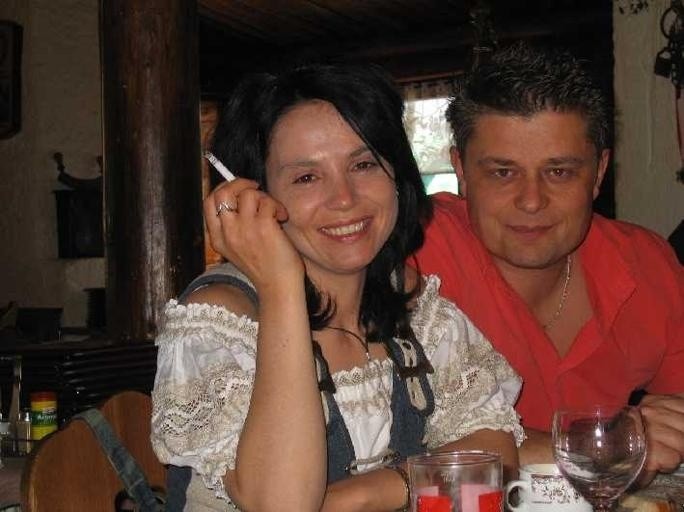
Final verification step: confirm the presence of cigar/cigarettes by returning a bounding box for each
[203,150,235,182]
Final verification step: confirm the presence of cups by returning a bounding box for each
[405,450,585,512]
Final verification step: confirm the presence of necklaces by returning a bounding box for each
[318,324,373,365]
[542,246,573,327]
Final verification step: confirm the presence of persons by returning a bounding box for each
[151,68,523,511]
[399,42,683,489]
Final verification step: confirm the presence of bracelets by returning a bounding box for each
[380,464,411,511]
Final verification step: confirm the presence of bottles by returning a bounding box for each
[29,391,58,440]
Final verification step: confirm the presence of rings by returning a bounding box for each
[213,200,235,212]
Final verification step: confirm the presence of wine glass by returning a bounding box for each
[551,406,646,511]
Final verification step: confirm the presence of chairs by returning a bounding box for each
[20,391,174,512]
